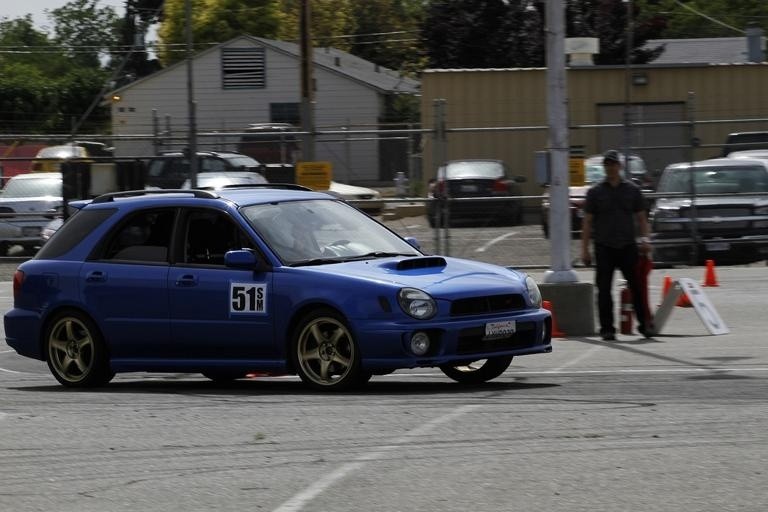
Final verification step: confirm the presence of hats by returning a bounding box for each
[604,150,620,160]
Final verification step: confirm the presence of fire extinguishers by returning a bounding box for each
[620,283,634,334]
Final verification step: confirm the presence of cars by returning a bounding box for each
[426,159,528,228]
[0,171,63,257]
[39,207,64,249]
[4,182,553,395]
[640,159,768,268]
[540,165,654,240]
[27,140,116,179]
[223,163,384,217]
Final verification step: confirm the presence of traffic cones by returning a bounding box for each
[542,300,565,337]
[657,276,675,309]
[700,259,719,286]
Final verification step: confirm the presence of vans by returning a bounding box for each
[141,149,263,195]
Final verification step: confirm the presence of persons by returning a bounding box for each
[580,148,656,343]
